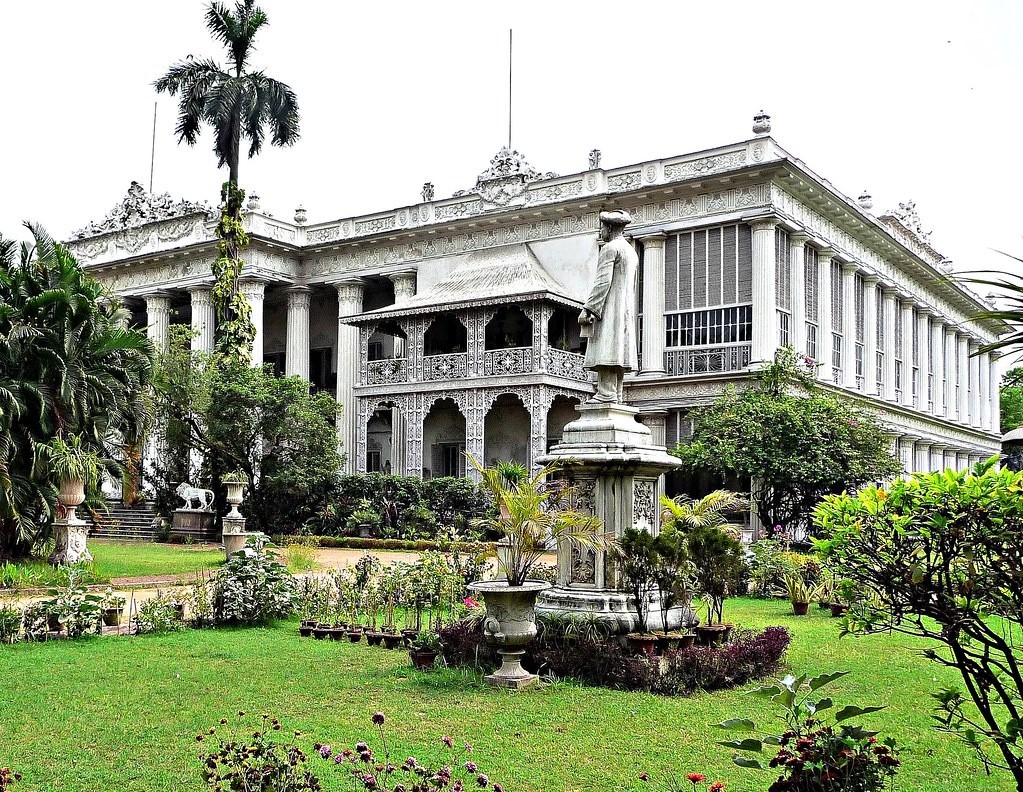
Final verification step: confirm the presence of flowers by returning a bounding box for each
[464,596,483,611]
[757,525,791,562]
[0,711,900,792]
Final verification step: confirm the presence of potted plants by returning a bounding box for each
[459,447,630,692]
[36,428,104,524]
[609,525,762,658]
[219,467,250,518]
[0,587,194,640]
[299,574,445,669]
[782,568,849,618]
